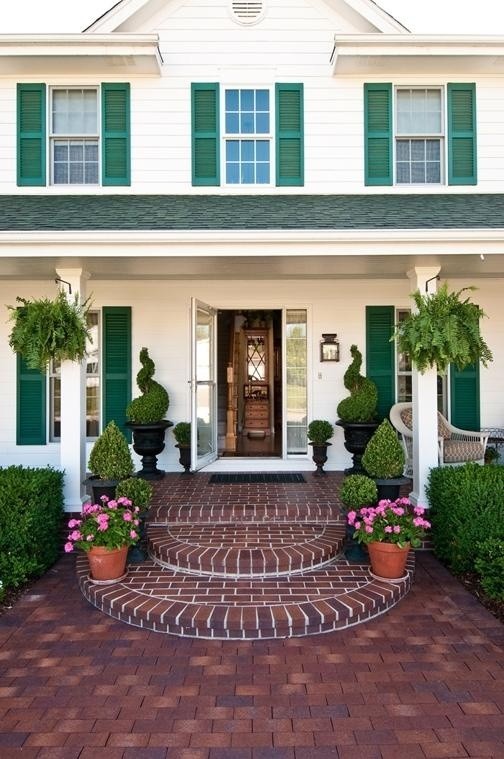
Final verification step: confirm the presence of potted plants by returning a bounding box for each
[111,475,154,566]
[332,344,414,505]
[169,419,200,479]
[302,414,333,478]
[337,473,380,564]
[123,341,176,482]
[78,418,133,506]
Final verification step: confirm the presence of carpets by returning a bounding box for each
[206,471,307,487]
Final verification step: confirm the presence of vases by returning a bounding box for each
[364,540,412,581]
[77,541,132,583]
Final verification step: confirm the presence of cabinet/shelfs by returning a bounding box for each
[244,329,268,396]
[246,400,270,429]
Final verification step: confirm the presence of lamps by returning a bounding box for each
[56,278,76,304]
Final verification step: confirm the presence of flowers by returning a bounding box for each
[344,494,434,555]
[58,493,141,555]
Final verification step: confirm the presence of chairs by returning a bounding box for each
[384,399,491,486]
